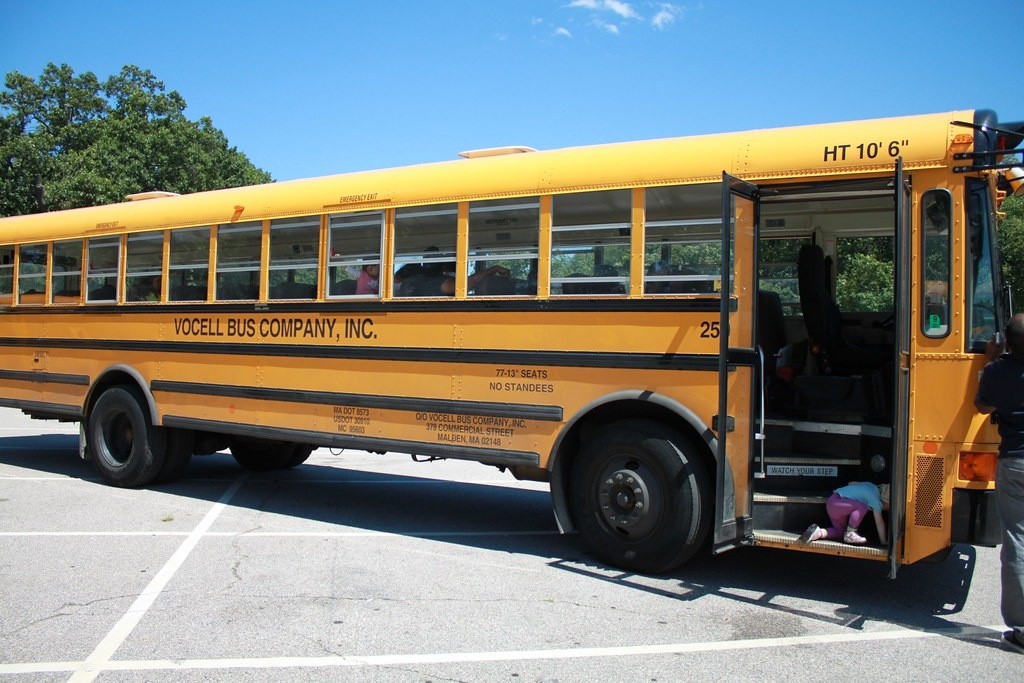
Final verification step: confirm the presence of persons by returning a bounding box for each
[802,482,891,545]
[974,314,1024,654]
[400,252,511,295]
[518,269,554,298]
[354,252,379,294]
[394,268,405,296]
[592,264,619,289]
[145,275,161,301]
[647,263,672,292]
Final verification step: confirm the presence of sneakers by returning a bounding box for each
[801,524,821,545]
[844,532,867,543]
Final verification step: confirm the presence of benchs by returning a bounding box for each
[20,273,786,362]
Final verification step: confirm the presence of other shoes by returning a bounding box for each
[1001,631,1024,654]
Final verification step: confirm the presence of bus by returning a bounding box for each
[0,105,1024,580]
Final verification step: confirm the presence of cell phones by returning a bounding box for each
[995,332,1001,346]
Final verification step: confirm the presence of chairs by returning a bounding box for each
[799,244,896,354]
